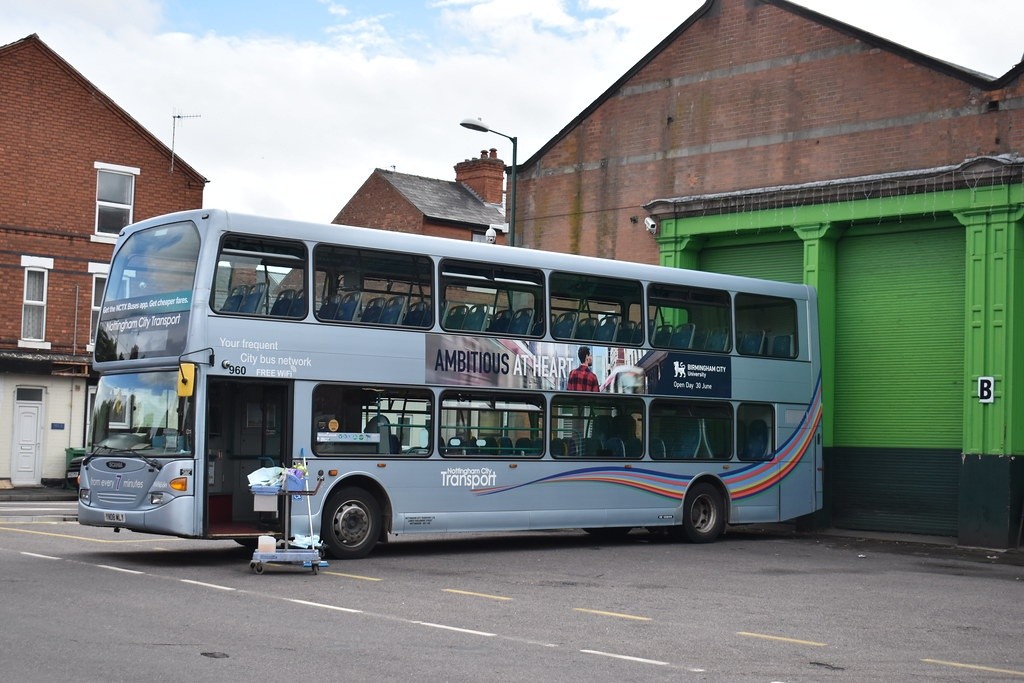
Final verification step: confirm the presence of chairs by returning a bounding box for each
[532,437,543,455]
[584,439,604,457]
[426,434,446,454]
[446,436,465,454]
[624,437,643,458]
[721,418,748,458]
[604,437,626,458]
[480,437,499,455]
[389,433,402,454]
[466,436,479,455]
[552,437,568,456]
[649,436,667,459]
[219,282,796,360]
[514,438,532,456]
[743,419,770,459]
[496,437,513,455]
[561,437,579,456]
[670,416,714,458]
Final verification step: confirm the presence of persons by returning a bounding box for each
[567,347,600,393]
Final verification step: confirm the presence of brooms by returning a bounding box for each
[300,447,329,567]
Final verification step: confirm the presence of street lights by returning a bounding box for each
[459,117,518,311]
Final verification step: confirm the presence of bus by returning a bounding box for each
[78,208,824,560]
